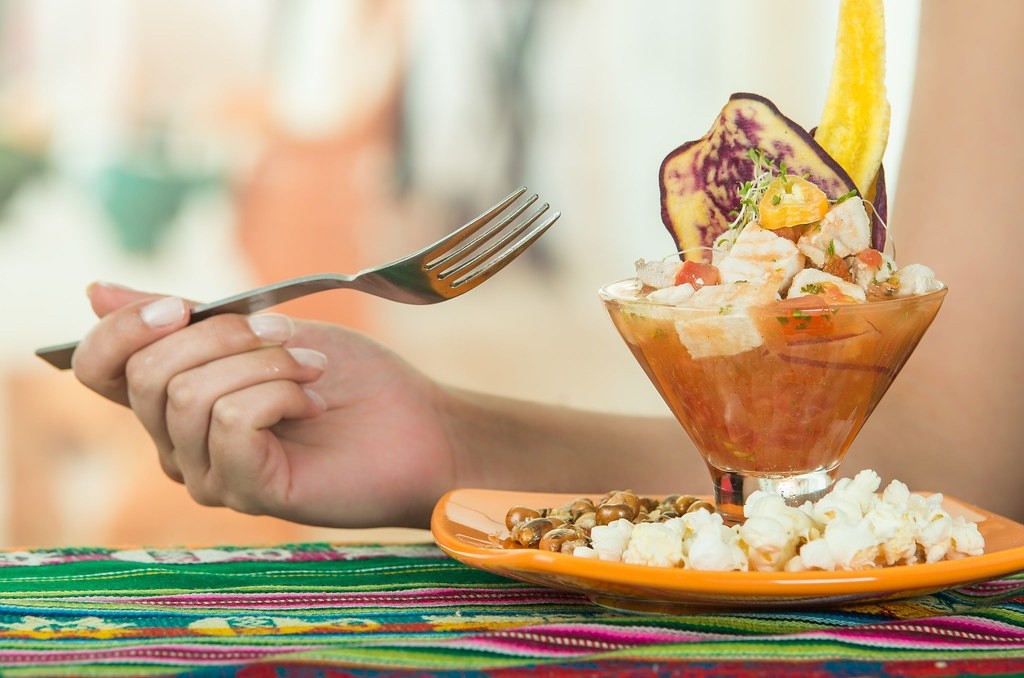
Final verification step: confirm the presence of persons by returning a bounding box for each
[67,0,1024,544]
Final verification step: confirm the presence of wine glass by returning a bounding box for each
[600,279,947,531]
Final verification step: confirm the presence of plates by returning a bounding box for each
[430,487,1023,609]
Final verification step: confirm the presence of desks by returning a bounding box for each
[0,541,1024,678]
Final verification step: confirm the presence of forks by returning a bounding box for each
[34,186,560,372]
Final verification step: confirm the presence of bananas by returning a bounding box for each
[623,194,942,357]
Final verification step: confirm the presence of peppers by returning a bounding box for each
[757,175,828,230]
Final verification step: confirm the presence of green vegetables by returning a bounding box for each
[660,154,898,329]
